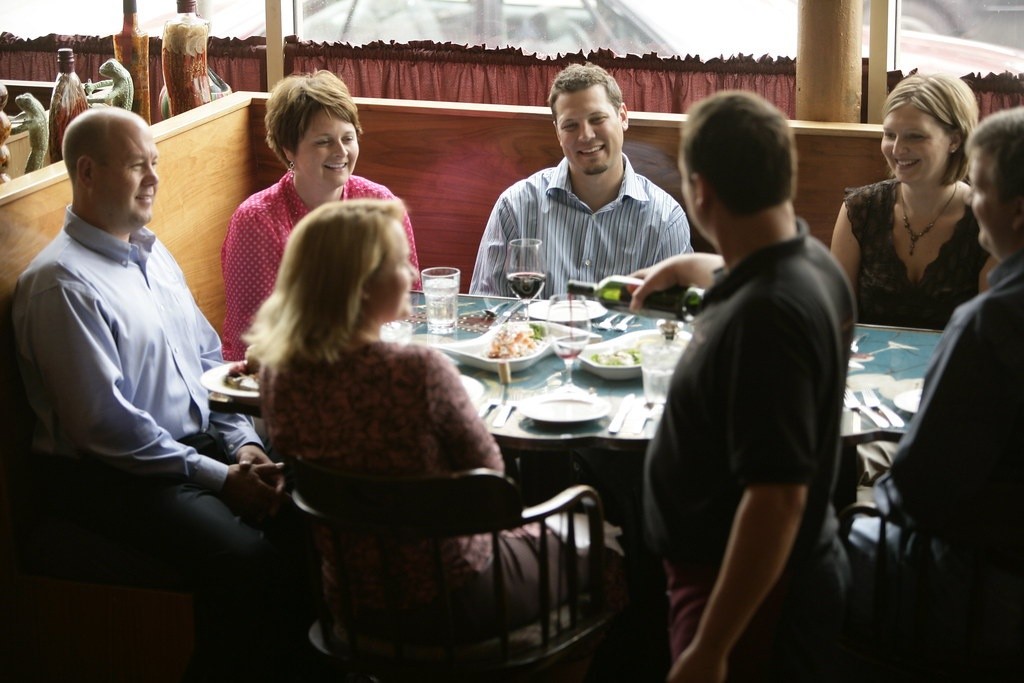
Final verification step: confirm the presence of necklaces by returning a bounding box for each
[900,180,957,256]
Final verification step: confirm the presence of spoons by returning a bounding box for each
[483,302,510,316]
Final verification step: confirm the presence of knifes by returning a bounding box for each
[607,393,635,433]
[489,301,527,328]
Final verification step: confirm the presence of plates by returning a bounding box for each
[521,391,611,423]
[200,360,260,397]
[460,374,484,405]
[430,321,603,373]
[577,329,693,379]
[524,300,606,323]
[893,390,923,414]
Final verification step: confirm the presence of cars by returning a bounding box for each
[117,0,1024,77]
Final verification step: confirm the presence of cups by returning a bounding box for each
[421,267,461,335]
[380,320,413,345]
[640,342,682,415]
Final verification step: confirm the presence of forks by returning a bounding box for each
[610,315,635,331]
[861,389,905,429]
[492,389,523,427]
[593,313,621,328]
[850,333,867,352]
[477,389,503,418]
[842,387,890,428]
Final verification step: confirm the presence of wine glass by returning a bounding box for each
[546,294,592,390]
[505,239,546,322]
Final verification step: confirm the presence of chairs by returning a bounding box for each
[289,454,948,683]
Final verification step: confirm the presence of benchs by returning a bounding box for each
[0,91,895,683]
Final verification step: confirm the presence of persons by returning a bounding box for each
[221,70,423,361]
[624,88,850,683]
[848,105,1024,613]
[240,197,585,656]
[467,62,694,300]
[829,72,998,333]
[10,105,307,682]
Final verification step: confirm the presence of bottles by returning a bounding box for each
[566,275,705,324]
[49,48,88,165]
[161,0,212,117]
[113,0,150,128]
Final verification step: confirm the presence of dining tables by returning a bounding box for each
[209,290,949,506]
